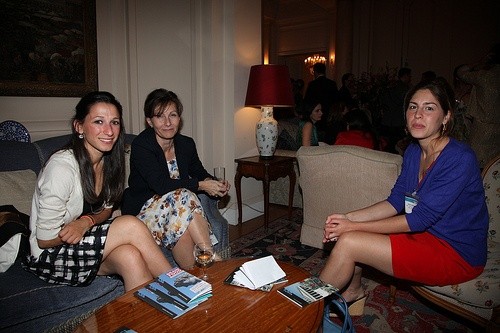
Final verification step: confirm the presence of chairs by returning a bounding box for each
[295,143,500,333]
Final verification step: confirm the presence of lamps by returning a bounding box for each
[245,63,296,161]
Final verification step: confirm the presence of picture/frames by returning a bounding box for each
[0,0,99,98]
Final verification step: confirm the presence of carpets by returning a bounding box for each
[229,212,486,333]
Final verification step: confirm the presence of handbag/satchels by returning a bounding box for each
[322,293,356,333]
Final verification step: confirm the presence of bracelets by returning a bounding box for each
[81,215,94,224]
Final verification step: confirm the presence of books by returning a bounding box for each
[223,266,288,292]
[154,267,213,304]
[277,276,339,308]
[133,287,198,319]
[144,282,208,310]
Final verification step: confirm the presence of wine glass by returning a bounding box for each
[193,241,215,280]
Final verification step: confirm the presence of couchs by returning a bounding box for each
[0,133,232,333]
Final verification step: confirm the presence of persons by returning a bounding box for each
[382,67,411,154]
[422,70,436,80]
[338,73,354,110]
[29,91,173,294]
[304,63,337,146]
[319,77,490,316]
[121,88,232,267]
[462,43,500,167]
[334,109,387,151]
[454,68,472,110]
[297,100,323,151]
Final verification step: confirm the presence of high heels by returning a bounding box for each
[328,295,366,317]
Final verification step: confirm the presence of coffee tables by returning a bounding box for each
[233,154,296,233]
[68,258,325,333]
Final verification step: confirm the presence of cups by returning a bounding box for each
[214,167,225,196]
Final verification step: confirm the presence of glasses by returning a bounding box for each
[153,113,176,120]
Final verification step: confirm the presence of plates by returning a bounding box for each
[0,120,31,142]
